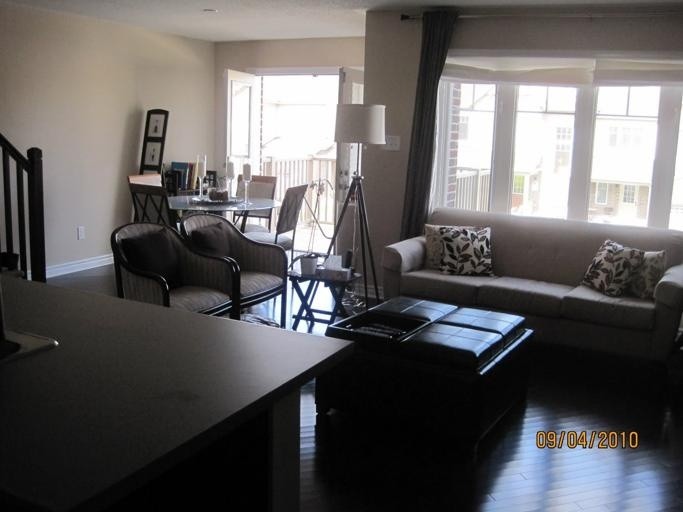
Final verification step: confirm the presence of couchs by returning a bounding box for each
[382,203,682,362]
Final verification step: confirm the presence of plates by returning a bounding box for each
[192,197,235,204]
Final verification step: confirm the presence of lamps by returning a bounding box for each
[303,103,387,330]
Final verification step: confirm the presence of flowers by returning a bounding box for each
[303,178,333,253]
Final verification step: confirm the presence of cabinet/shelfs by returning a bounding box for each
[165,169,218,222]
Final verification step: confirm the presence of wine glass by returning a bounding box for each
[226,156,235,201]
[195,155,207,198]
[240,158,253,205]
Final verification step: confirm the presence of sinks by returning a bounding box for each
[0,328,59,365]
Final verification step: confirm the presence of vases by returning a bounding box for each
[299,254,318,277]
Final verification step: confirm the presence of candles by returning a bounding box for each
[197,161,205,176]
[226,161,234,177]
[243,164,250,181]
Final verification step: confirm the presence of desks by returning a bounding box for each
[166,195,278,226]
[285,268,362,336]
[0,268,355,512]
[324,292,535,480]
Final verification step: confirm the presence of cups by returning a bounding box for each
[300,257,317,275]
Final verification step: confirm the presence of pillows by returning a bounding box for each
[630,249,667,299]
[423,221,479,270]
[189,219,232,258]
[438,224,495,278]
[580,237,645,296]
[119,227,183,290]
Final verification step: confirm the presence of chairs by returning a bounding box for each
[175,212,290,327]
[125,173,161,187]
[230,174,276,235]
[129,181,172,228]
[241,183,309,269]
[110,224,240,318]
